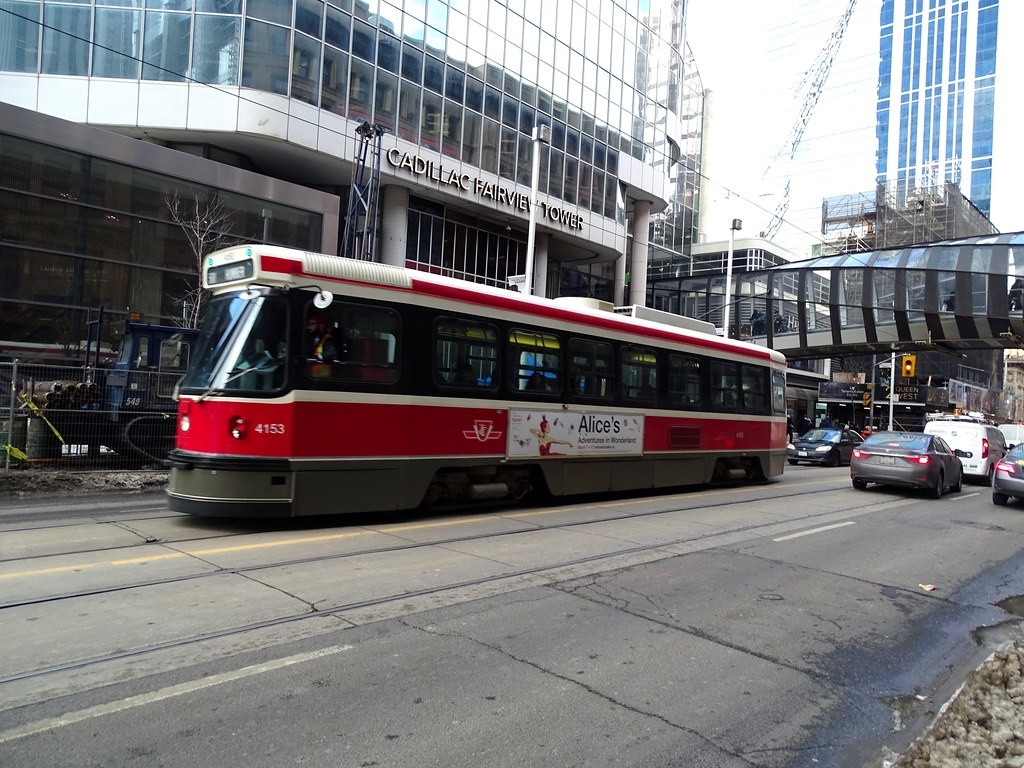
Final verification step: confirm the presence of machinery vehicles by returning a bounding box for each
[0,319,204,460]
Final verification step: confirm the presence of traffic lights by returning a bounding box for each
[902,355,916,377]
[863,394,871,407]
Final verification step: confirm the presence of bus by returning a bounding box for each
[165,244,790,517]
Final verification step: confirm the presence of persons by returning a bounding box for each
[1008,278,1023,310]
[749,310,787,336]
[308,317,335,377]
[801,414,861,436]
[787,414,794,444]
[943,291,956,311]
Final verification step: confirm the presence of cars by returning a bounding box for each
[992,443,1024,505]
[850,430,964,499]
[786,427,866,467]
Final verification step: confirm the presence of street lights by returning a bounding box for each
[722,219,744,338]
[523,124,551,295]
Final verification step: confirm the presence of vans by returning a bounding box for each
[923,409,1014,487]
[998,420,1024,451]
